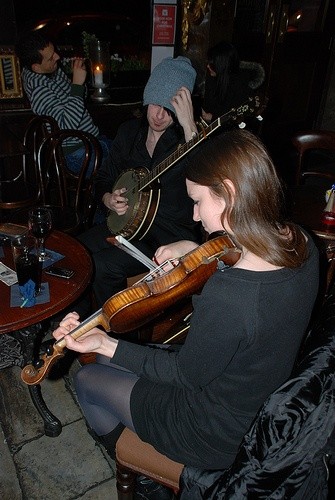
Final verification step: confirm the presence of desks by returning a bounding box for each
[0,223,95,437]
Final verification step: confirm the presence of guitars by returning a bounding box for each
[106,95,267,240]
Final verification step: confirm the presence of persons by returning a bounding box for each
[17,30,111,176]
[193,42,252,127]
[40,55,206,381]
[52,127,320,500]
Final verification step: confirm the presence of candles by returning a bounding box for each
[94,67,103,86]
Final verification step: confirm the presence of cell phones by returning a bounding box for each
[45,266,74,279]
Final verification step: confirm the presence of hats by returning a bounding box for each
[142,56,198,118]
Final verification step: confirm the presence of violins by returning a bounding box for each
[21,232,242,385]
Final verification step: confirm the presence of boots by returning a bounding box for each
[97,421,178,499]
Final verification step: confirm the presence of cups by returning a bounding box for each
[12,236,43,298]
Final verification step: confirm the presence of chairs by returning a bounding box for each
[0,115,102,237]
[115,425,184,500]
[285,130,335,290]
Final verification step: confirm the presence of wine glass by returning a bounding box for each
[28,208,51,261]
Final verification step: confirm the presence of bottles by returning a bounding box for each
[127,58,131,65]
[61,58,89,72]
[137,57,144,70]
